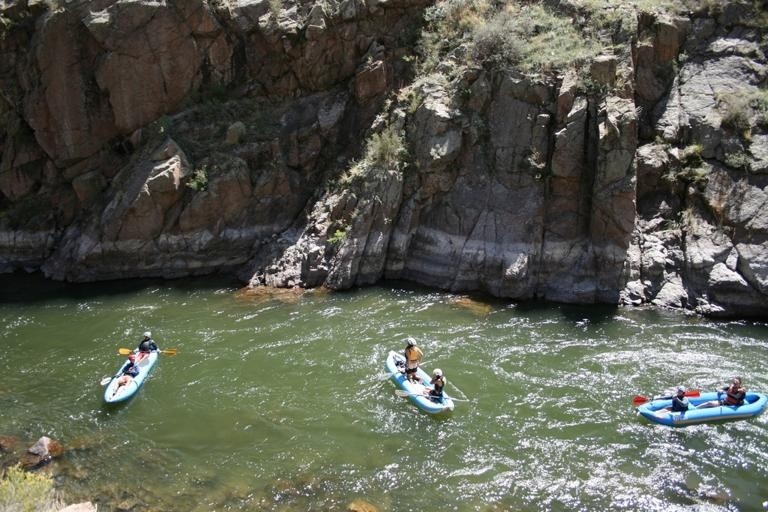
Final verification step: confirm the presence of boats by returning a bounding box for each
[386,350,455,414]
[103,346,158,404]
[636,391,768,429]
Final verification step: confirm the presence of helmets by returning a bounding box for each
[734,378,741,384]
[143,332,151,338]
[128,355,136,360]
[408,338,417,346]
[676,386,686,392]
[433,368,443,377]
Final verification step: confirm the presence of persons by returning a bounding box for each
[694,374,748,409]
[403,336,425,385]
[422,367,449,405]
[117,354,141,385]
[653,384,688,413]
[137,331,158,359]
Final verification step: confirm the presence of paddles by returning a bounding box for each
[378,360,433,381]
[632,389,701,404]
[100,354,148,386]
[118,347,178,357]
[395,389,470,402]
